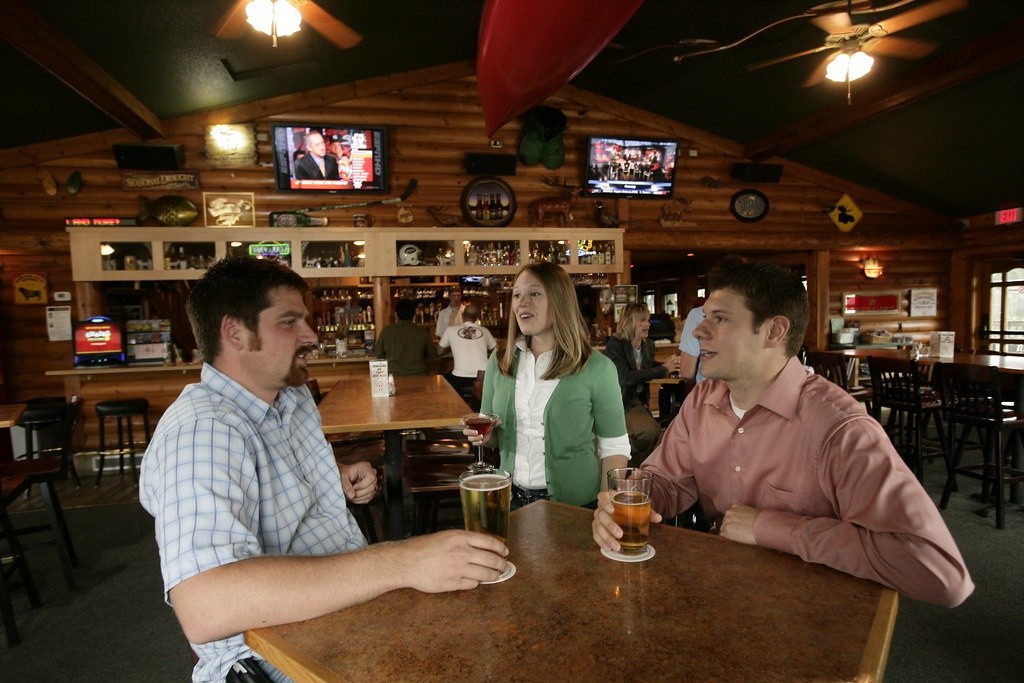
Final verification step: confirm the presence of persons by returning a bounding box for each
[460,259,632,516]
[592,260,977,611]
[138,256,511,683]
[678,306,711,386]
[376,298,435,375]
[435,286,498,392]
[603,303,681,480]
[589,140,664,184]
[288,126,355,182]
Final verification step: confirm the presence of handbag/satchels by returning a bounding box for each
[683,380,696,400]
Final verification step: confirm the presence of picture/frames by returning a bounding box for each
[730,188,769,224]
[460,177,521,227]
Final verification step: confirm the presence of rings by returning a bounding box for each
[675,360,676,362]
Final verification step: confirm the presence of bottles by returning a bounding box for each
[301,243,355,268]
[388,373,396,395]
[578,244,616,265]
[393,286,509,330]
[268,212,327,227]
[304,288,380,360]
[528,240,569,264]
[176,246,216,271]
[436,248,447,266]
[463,242,522,266]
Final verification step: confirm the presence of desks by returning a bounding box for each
[240,498,900,683]
[313,375,475,542]
[0,404,25,428]
[831,344,1024,505]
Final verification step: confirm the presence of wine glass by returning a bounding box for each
[460,413,500,470]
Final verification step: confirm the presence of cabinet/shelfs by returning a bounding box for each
[72,224,625,372]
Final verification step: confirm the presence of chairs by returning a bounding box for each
[808,347,1024,529]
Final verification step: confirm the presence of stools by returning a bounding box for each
[0,396,478,646]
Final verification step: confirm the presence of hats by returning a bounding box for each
[448,285,461,294]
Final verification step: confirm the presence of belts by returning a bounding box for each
[512,483,548,502]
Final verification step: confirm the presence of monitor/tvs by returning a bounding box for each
[269,121,391,194]
[583,135,680,199]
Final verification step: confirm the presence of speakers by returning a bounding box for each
[464,152,517,177]
[113,141,186,169]
[730,161,785,183]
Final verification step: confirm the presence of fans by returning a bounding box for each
[214,0,363,50]
[746,0,969,88]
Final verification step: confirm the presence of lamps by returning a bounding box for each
[824,39,875,102]
[246,0,301,48]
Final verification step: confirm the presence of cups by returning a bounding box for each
[457,468,512,576]
[353,214,368,228]
[606,467,655,559]
